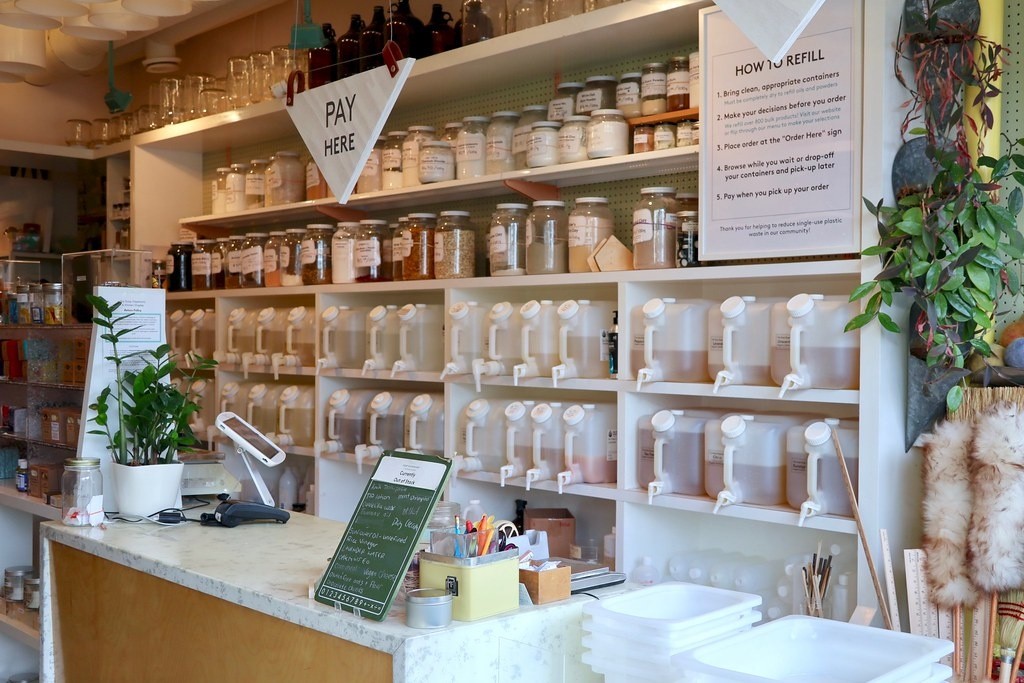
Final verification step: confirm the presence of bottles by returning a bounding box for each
[630,542,857,624]
[4,565,40,610]
[60,457,103,528]
[62,1,702,271]
[425,501,462,552]
[16,459,29,492]
[0,284,63,326]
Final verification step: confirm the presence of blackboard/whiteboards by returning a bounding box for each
[314,449,454,621]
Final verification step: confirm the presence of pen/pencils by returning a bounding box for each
[464,515,493,558]
[455,514,460,557]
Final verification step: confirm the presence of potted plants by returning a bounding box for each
[82,293,217,521]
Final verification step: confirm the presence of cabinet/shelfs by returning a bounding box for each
[0,0,945,624]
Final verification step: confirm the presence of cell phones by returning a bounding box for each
[215,412,286,467]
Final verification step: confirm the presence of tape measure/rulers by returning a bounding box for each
[904,549,939,638]
[880,528,901,631]
[939,603,952,669]
[970,591,991,683]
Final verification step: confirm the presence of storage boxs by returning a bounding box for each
[417,548,519,622]
[581,580,955,683]
[60,248,151,327]
[519,567,571,605]
[523,508,575,559]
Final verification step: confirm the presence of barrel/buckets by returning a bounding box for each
[170,295,860,527]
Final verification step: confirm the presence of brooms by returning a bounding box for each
[997,588,1024,683]
